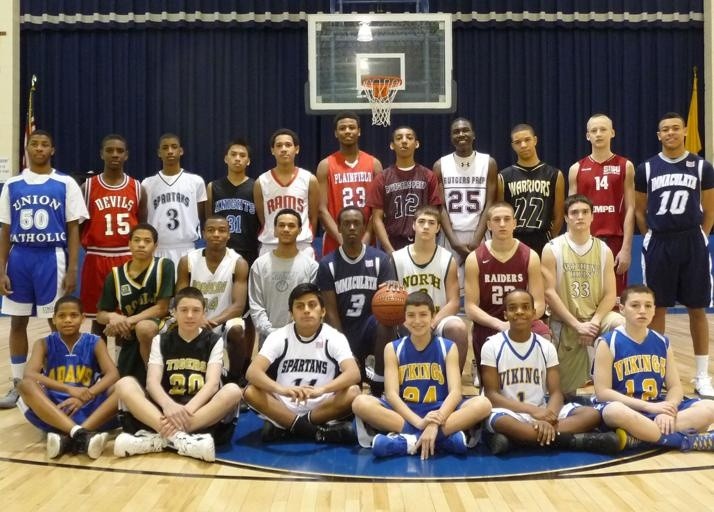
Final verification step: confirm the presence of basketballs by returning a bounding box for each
[372,286,409,328]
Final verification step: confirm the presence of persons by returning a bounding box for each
[353,291,493,457]
[252,209,320,345]
[92,225,176,371]
[633,109,712,400]
[178,216,250,374]
[18,296,123,460]
[0,122,84,409]
[389,205,468,377]
[77,132,147,344]
[465,200,556,386]
[541,191,633,389]
[592,283,713,455]
[115,286,244,462]
[319,205,401,395]
[139,110,637,312]
[479,286,598,457]
[246,282,362,443]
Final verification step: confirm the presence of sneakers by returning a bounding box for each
[693,377,714,399]
[0,378,21,409]
